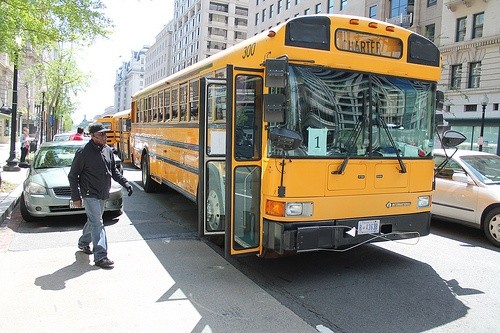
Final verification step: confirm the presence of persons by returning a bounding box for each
[477,160,487,176]
[67,122,133,267]
[20,127,30,162]
[216,107,222,119]
[68,126,84,141]
[83,131,91,137]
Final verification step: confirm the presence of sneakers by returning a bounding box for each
[95,256,114,265]
[78,245,93,254]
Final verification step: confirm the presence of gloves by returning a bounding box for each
[126,185,134,196]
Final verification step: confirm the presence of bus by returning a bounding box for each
[97,115,114,146]
[113,110,139,169]
[130,14,443,260]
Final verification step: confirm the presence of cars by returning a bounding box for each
[20,141,122,222]
[53,135,79,142]
[430,149,500,248]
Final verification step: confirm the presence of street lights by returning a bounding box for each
[6,48,33,170]
[478,94,489,153]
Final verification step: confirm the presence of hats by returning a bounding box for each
[89,122,111,133]
